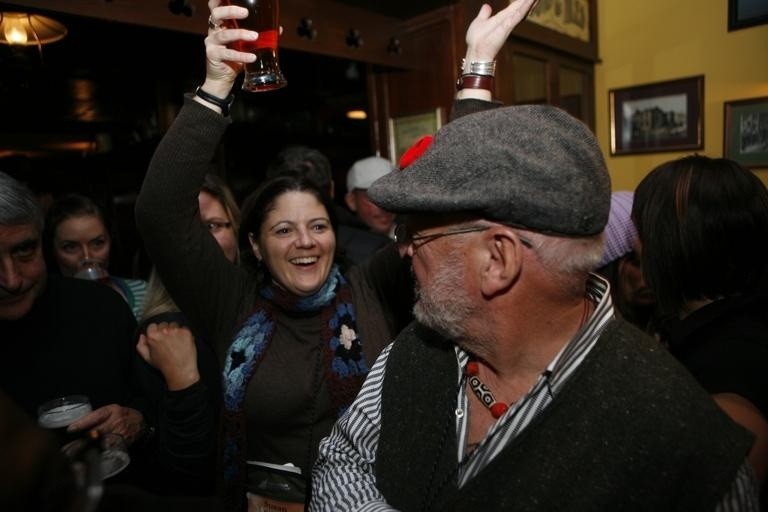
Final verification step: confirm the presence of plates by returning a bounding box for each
[102,450,130,481]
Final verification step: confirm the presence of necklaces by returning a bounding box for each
[466,361,509,420]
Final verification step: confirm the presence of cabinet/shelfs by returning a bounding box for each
[365,0,598,153]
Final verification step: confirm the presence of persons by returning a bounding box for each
[630,152,768,512]
[44,191,149,324]
[304,104,760,512]
[345,155,400,242]
[265,142,395,277]
[132,0,539,511]
[0,170,139,488]
[131,169,242,497]
[592,188,659,330]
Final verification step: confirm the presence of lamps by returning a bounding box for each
[0,0,69,54]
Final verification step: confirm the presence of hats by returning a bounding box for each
[366,104,610,237]
[346,157,393,192]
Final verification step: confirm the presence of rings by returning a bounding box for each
[209,16,225,31]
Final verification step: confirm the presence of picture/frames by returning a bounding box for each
[605,71,706,160]
[725,1,768,34]
[720,94,768,170]
[385,105,445,172]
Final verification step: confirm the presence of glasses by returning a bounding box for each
[394,224,532,264]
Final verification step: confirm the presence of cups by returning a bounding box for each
[38,399,103,478]
[71,260,111,286]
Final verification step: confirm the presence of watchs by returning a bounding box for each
[194,85,235,118]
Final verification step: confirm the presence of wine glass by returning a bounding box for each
[226,1,287,93]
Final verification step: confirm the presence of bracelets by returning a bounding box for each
[460,56,496,77]
[455,75,497,94]
